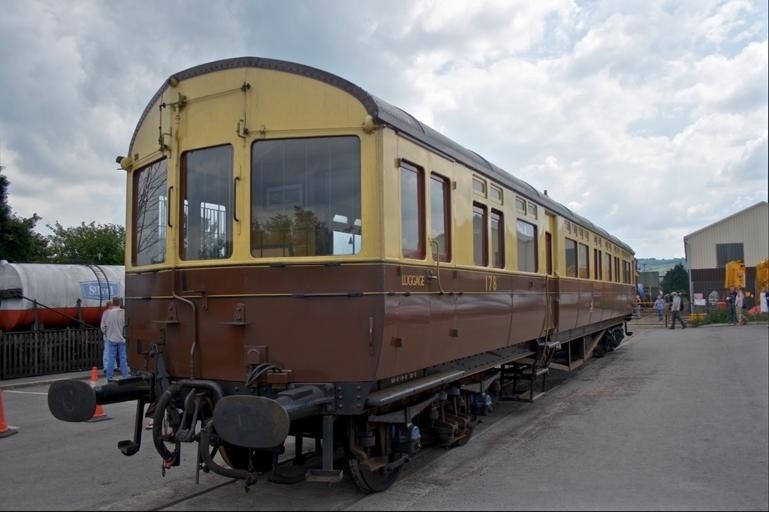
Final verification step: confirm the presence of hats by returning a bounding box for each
[672,292,677,295]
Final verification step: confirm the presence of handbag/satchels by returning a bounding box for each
[743,304,746,308]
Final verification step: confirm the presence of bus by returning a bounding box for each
[48,56,639,494]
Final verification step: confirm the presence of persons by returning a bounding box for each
[102,296,130,382]
[726,284,737,325]
[667,292,687,329]
[734,287,746,325]
[101,301,119,373]
[652,293,666,322]
[635,294,642,319]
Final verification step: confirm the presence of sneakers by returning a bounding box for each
[670,326,674,329]
[682,326,686,328]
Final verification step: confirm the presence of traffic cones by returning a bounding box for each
[86,367,113,422]
[0,384,18,438]
